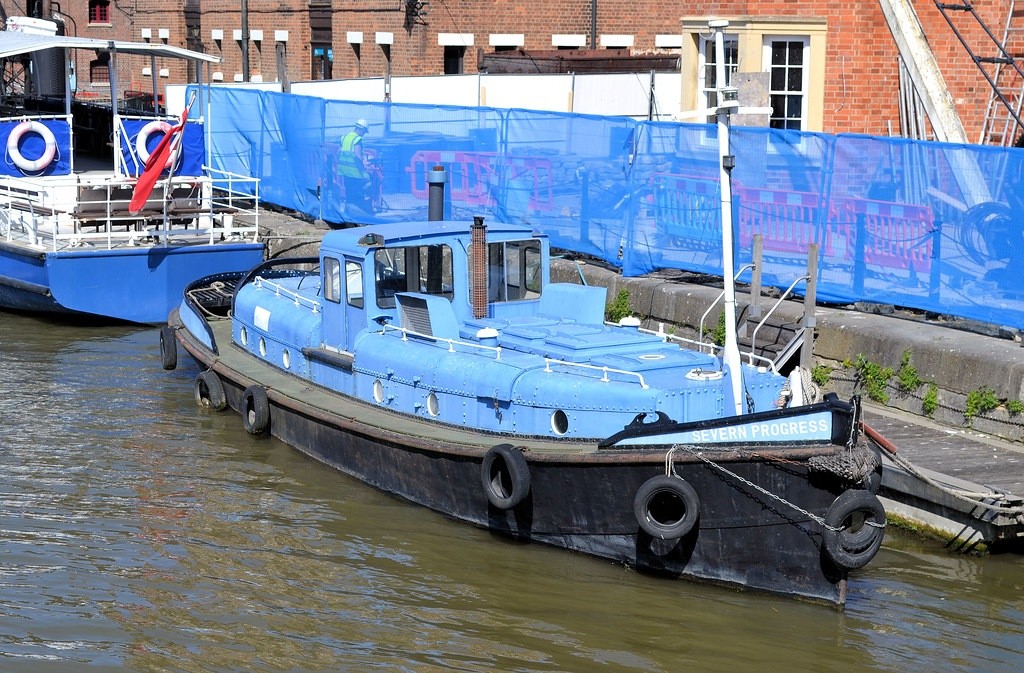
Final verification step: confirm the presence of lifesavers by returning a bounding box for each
[193,370,227,412]
[134,120,183,169]
[823,489,886,570]
[241,385,269,435]
[633,475,700,540]
[481,443,531,510]
[6,121,56,172]
[160,327,177,370]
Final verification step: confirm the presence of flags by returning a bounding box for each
[129,109,189,212]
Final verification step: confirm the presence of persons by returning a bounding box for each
[339,119,371,213]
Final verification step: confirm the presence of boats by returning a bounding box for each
[167,21,864,612]
[0,16,265,327]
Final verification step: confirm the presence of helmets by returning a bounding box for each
[355,119,369,134]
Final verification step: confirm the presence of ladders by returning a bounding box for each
[973,1,1024,205]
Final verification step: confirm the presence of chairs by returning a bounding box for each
[77,188,198,232]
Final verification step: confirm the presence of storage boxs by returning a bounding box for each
[459,316,713,372]
